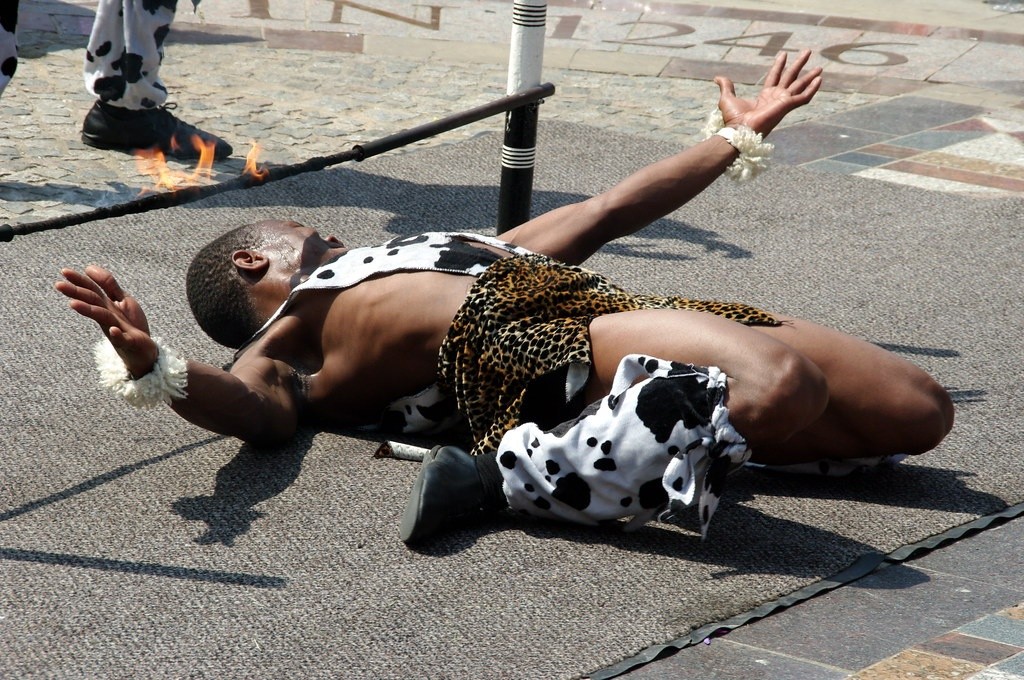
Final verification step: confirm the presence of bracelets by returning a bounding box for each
[713,124,775,184]
[93,334,189,410]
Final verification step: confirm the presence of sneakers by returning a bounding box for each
[402,444,501,547]
[82,102,232,163]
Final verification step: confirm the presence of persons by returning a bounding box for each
[0,0,234,161]
[55,47,955,542]
